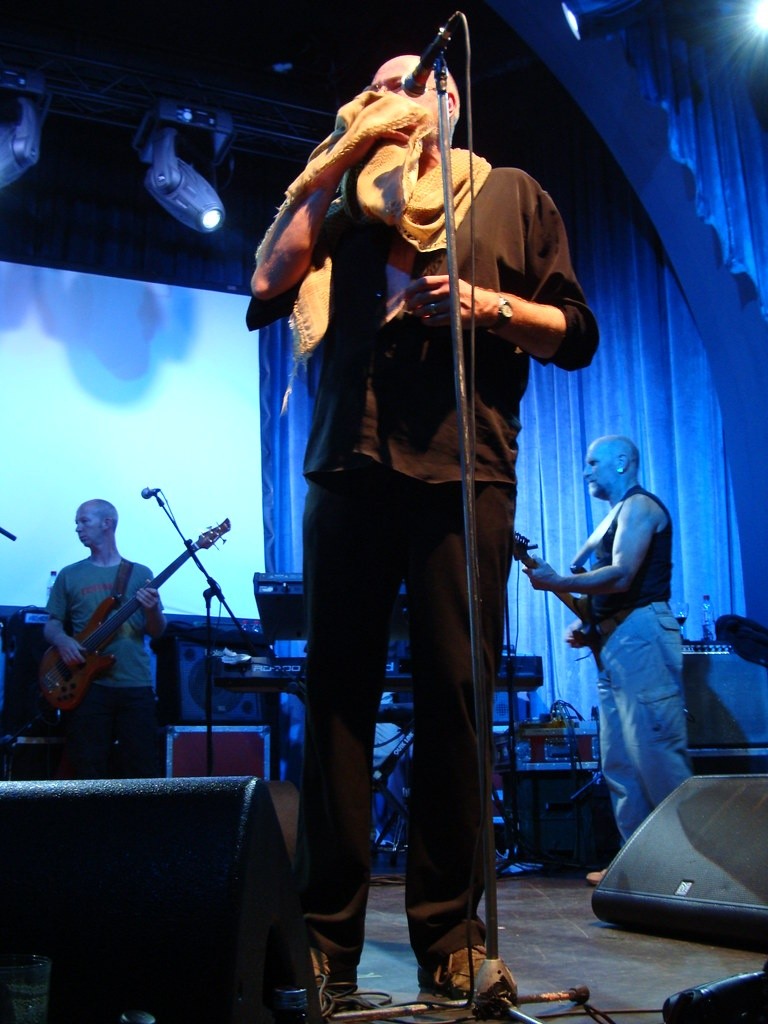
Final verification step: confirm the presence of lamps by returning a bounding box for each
[133,103,233,231]
[1,77,47,188]
[562,0,643,42]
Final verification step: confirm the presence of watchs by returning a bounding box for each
[476,292,513,334]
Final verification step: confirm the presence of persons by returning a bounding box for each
[43,499,166,778]
[522,434,693,887]
[251,54,599,999]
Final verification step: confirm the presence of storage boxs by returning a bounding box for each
[520,728,599,762]
[165,725,272,781]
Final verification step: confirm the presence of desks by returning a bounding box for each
[499,761,598,874]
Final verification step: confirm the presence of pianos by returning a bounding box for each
[213,572,545,873]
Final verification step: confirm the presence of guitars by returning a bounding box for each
[510,532,606,671]
[42,518,232,710]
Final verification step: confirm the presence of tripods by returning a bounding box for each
[325,58,590,1024]
[495,588,583,876]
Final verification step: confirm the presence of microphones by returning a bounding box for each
[140,486,161,499]
[401,10,462,98]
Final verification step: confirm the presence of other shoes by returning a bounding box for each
[587,870,606,886]
[416,945,488,999]
[312,950,358,993]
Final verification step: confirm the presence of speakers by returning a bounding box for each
[0,775,323,1024]
[590,774,768,947]
[2,607,75,738]
[161,640,262,724]
[681,639,768,748]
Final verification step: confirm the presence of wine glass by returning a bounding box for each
[672,602,689,641]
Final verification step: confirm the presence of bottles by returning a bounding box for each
[120,1011,157,1024]
[700,595,717,641]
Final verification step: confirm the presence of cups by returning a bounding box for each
[0,955,52,1024]
